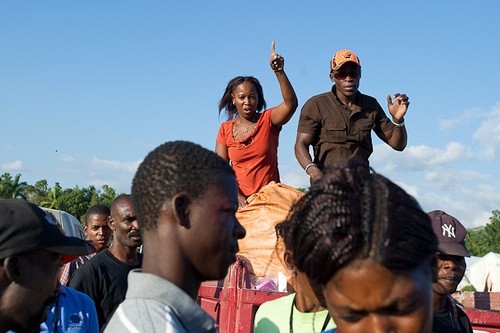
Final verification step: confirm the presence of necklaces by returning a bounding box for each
[237,112,258,133]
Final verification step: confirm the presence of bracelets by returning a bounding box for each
[391,116,405,126]
[305,164,317,175]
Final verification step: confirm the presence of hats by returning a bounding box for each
[0,198,92,262]
[427,210,471,258]
[40,207,96,257]
[330,50,361,73]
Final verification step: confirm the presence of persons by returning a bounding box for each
[215,40,298,209]
[294,49,410,186]
[0,193,144,333]
[102,141,246,333]
[253,163,473,333]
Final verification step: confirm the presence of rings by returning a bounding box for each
[243,200,248,204]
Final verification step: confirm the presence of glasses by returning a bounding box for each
[331,70,360,80]
